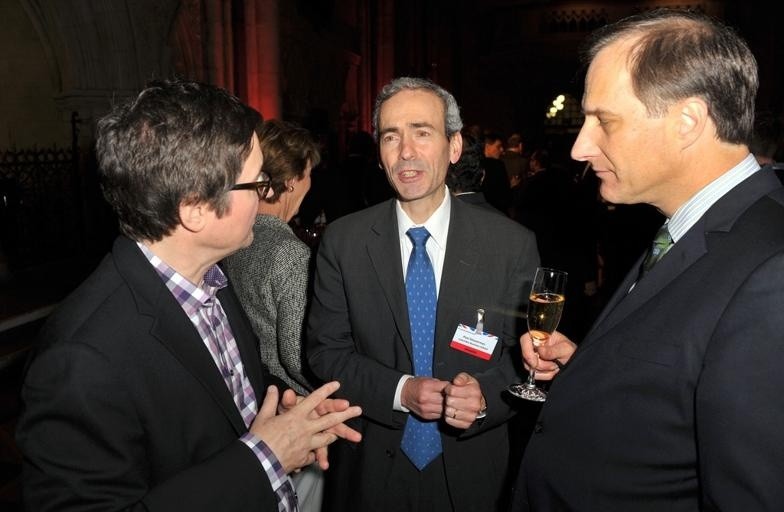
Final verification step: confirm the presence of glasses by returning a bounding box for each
[230,170,272,200]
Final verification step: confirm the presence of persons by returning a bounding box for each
[4,78,362,510]
[299,77,541,512]
[751,111,781,182]
[450,126,663,341]
[514,18,783,510]
[224,119,331,395]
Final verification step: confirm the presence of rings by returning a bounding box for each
[453,409,458,418]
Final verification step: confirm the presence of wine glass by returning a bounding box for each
[507,268,569,406]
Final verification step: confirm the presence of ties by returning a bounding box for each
[636,223,674,286]
[400,226,443,471]
